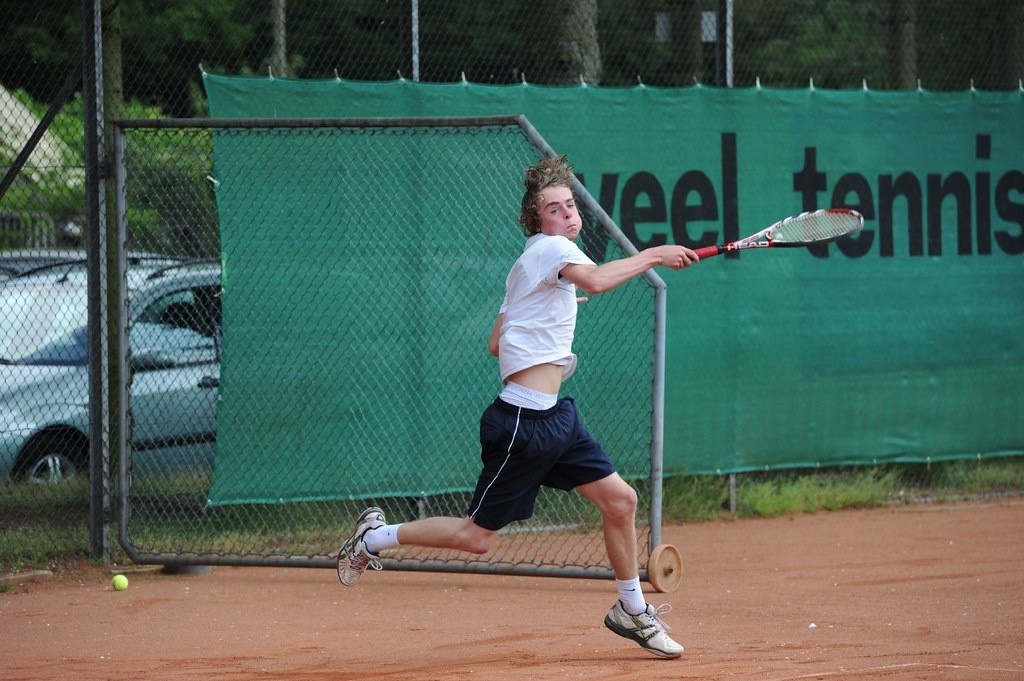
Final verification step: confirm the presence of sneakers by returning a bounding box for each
[605,600,685,659]
[337,508,387,586]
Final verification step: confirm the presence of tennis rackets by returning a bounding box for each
[687,208,865,261]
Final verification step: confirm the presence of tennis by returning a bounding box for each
[111,574,128,591]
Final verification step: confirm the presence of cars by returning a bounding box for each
[0,256,219,483]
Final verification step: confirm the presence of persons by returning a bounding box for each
[334,148,700,658]
